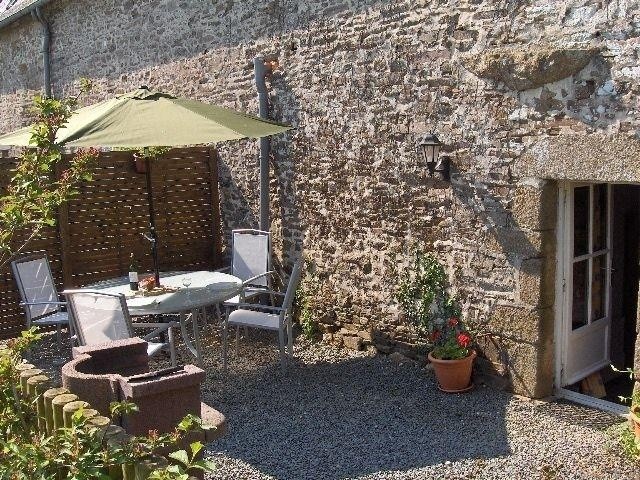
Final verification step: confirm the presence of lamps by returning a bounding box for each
[420,128,448,180]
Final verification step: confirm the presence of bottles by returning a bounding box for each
[128,253,138,291]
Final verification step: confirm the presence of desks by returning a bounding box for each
[69,269,247,380]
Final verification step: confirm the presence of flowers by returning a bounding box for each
[395,256,479,361]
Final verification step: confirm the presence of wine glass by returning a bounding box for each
[182,277,193,297]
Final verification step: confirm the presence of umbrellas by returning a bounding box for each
[0,85,297,342]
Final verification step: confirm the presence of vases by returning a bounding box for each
[422,339,486,397]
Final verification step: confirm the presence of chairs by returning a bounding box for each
[61,285,182,379]
[2,249,80,379]
[221,253,308,388]
[215,226,284,382]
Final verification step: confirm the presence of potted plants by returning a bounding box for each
[609,361,640,440]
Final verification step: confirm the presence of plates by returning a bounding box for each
[125,298,158,307]
